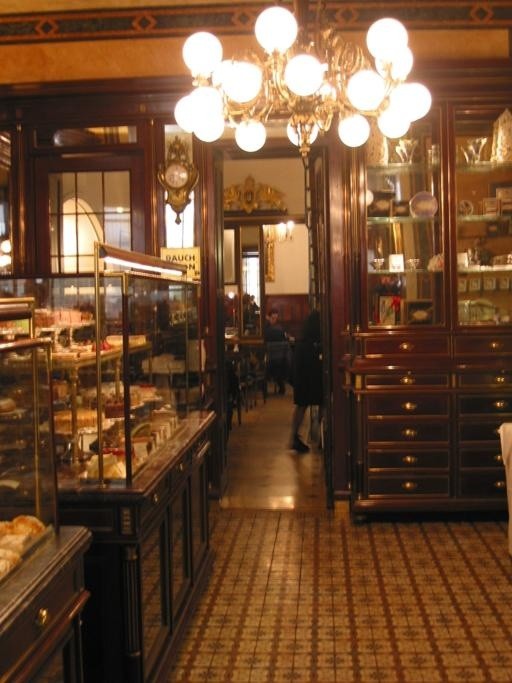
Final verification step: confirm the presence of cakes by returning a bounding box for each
[52,381,178,479]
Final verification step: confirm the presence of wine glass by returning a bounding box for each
[373,258,385,270]
[409,258,420,269]
[460,137,487,164]
[396,139,418,163]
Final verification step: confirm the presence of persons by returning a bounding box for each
[264,305,295,395]
[288,306,322,453]
[244,292,258,326]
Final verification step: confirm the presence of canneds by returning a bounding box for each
[34,308,50,327]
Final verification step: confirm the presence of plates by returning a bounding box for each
[409,190,437,217]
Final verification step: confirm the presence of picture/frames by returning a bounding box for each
[371,289,401,327]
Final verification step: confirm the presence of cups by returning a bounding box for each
[457,252,469,270]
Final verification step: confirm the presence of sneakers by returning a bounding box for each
[288,437,309,452]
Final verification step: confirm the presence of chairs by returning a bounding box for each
[218,331,293,427]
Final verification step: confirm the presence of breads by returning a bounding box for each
[50,309,94,324]
[0,515,46,576]
[1,398,15,412]
[107,335,146,347]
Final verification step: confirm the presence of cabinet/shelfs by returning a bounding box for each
[346,332,511,526]
[0,267,218,681]
[341,66,512,331]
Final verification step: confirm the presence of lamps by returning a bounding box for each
[94,242,190,279]
[168,2,431,165]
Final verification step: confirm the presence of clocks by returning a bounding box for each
[160,133,200,223]
